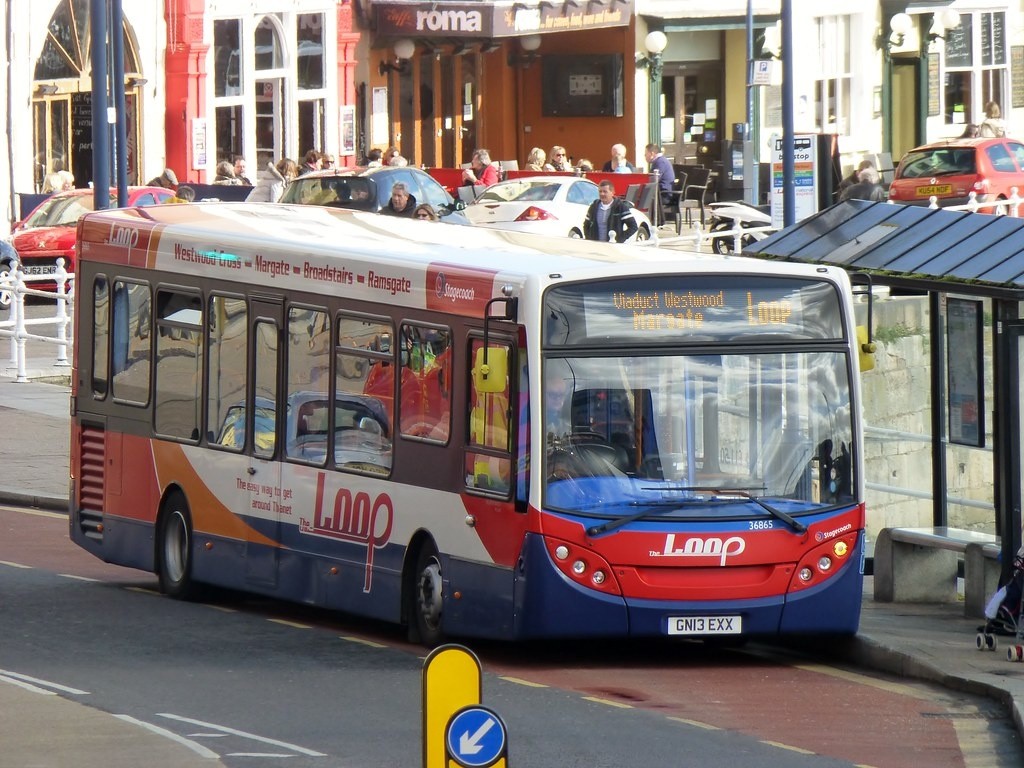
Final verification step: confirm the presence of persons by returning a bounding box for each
[244,158,297,203]
[959,101,1005,138]
[838,160,884,204]
[545,373,575,455]
[161,186,195,204]
[145,169,179,189]
[378,182,438,221]
[525,146,592,173]
[43,170,74,194]
[583,180,638,243]
[462,149,498,187]
[976,546,1023,637]
[645,144,674,229]
[362,146,408,167]
[296,149,335,205]
[603,143,637,173]
[212,156,251,186]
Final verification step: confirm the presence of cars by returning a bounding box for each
[277,167,467,220]
[441,176,659,248]
[887,137,1024,217]
[0,240,26,310]
[6,186,177,290]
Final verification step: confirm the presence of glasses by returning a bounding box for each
[549,393,564,399]
[417,214,427,217]
[325,160,334,164]
[557,154,565,156]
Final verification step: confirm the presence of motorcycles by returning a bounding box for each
[706,200,780,254]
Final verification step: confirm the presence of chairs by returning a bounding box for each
[618,165,711,236]
[458,160,519,203]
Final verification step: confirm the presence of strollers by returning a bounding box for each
[975,561,1024,662]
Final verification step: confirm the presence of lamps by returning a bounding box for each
[38,84,58,95]
[416,37,443,59]
[876,13,912,50]
[379,38,415,76]
[479,37,502,53]
[922,9,961,47]
[637,31,668,82]
[447,37,472,55]
[508,35,542,65]
[128,78,147,86]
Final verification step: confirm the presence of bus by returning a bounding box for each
[68,203,865,651]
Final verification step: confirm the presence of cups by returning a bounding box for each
[466,169,474,175]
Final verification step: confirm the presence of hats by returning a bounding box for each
[163,169,179,186]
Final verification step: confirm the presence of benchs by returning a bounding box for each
[874,527,1001,619]
